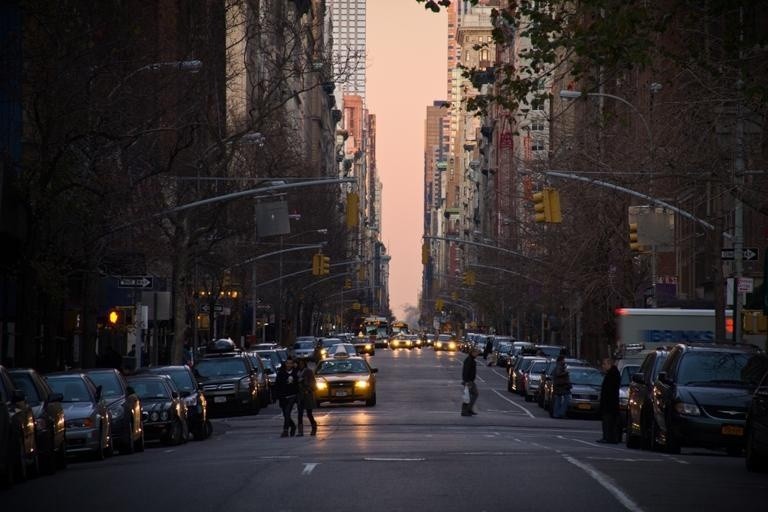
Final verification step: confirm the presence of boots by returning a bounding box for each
[310,421,317,435]
[296,423,303,437]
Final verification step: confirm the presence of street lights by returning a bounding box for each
[194,208,391,358]
[454,227,553,329]
[106,55,204,104]
[195,130,269,193]
[553,87,656,309]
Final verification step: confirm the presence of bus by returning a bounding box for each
[391,320,409,334]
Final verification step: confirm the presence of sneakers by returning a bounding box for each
[290,425,296,436]
[470,411,477,415]
[281,431,289,438]
[461,411,472,416]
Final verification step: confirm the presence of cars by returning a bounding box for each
[744,371,768,478]
[460,331,608,420]
[0,364,42,496]
[7,365,67,473]
[83,365,145,453]
[38,370,117,460]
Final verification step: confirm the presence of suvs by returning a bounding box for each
[650,337,768,474]
[602,342,649,444]
[622,345,671,451]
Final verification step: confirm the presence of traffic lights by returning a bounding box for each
[108,311,125,324]
[435,299,439,310]
[452,292,456,301]
[441,301,445,312]
[531,188,551,225]
[627,222,643,251]
[423,244,431,263]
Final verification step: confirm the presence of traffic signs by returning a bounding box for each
[721,247,760,262]
[115,275,155,291]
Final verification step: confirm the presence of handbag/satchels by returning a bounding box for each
[552,381,572,396]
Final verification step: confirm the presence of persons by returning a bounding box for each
[461,349,480,416]
[295,359,317,437]
[272,356,298,438]
[483,337,492,359]
[552,348,572,419]
[596,357,623,444]
